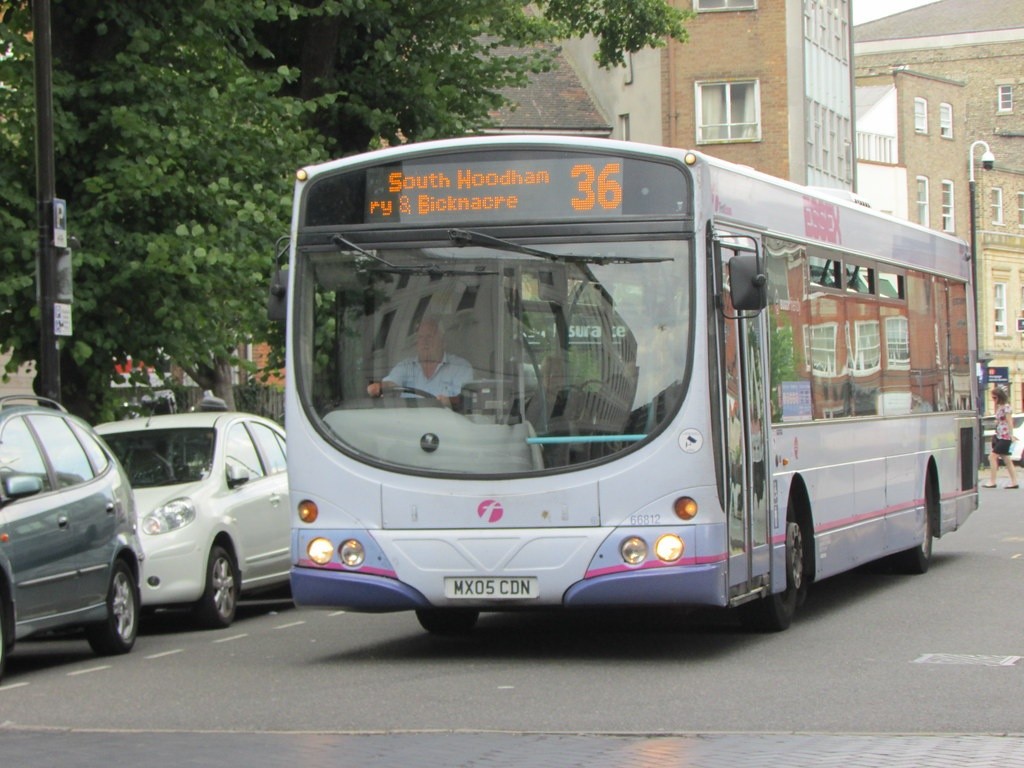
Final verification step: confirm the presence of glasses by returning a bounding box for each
[992,397,998,399]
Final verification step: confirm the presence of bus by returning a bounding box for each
[270,133,980,639]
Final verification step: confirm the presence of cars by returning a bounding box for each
[88,411,291,628]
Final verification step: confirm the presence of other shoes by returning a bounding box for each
[981,483,997,488]
[1003,484,1019,489]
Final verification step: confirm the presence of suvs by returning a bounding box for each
[982,415,1024,467]
[0,394,144,679]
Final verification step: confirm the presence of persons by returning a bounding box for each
[367,316,478,406]
[980,387,1019,489]
